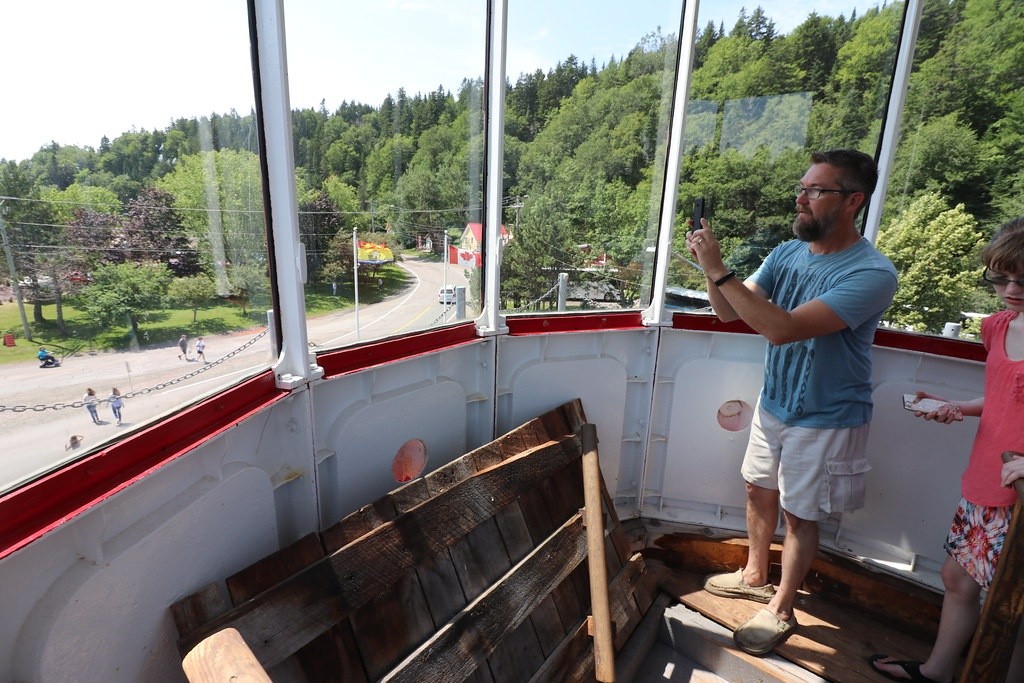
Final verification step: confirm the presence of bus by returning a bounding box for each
[541,268,624,302]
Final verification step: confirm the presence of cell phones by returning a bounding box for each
[692,197,705,234]
[903,393,963,422]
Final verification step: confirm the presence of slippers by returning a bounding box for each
[868,653,942,683]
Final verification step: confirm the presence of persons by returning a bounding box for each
[178,334,189,360]
[685,148,900,657]
[37,347,58,365]
[195,337,210,363]
[108,388,122,426]
[869,217,1024,683]
[378,277,383,291]
[82,387,101,424]
[64,435,85,452]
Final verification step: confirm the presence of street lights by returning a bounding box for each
[371,204,394,233]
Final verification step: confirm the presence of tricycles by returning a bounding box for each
[36,352,60,368]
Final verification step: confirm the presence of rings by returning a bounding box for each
[699,239,703,243]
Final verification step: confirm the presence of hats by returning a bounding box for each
[69,435,78,443]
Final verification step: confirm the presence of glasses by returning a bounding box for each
[982,266,1024,289]
[794,185,860,200]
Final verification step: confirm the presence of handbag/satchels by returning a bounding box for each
[197,350,203,354]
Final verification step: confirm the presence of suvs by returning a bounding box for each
[439,285,456,304]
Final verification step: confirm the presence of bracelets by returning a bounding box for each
[714,270,736,287]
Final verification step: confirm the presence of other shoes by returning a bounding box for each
[53,360,59,365]
[703,568,775,603]
[93,418,99,423]
[179,355,181,360]
[203,362,210,364]
[196,359,199,362]
[117,419,121,426]
[733,607,799,655]
